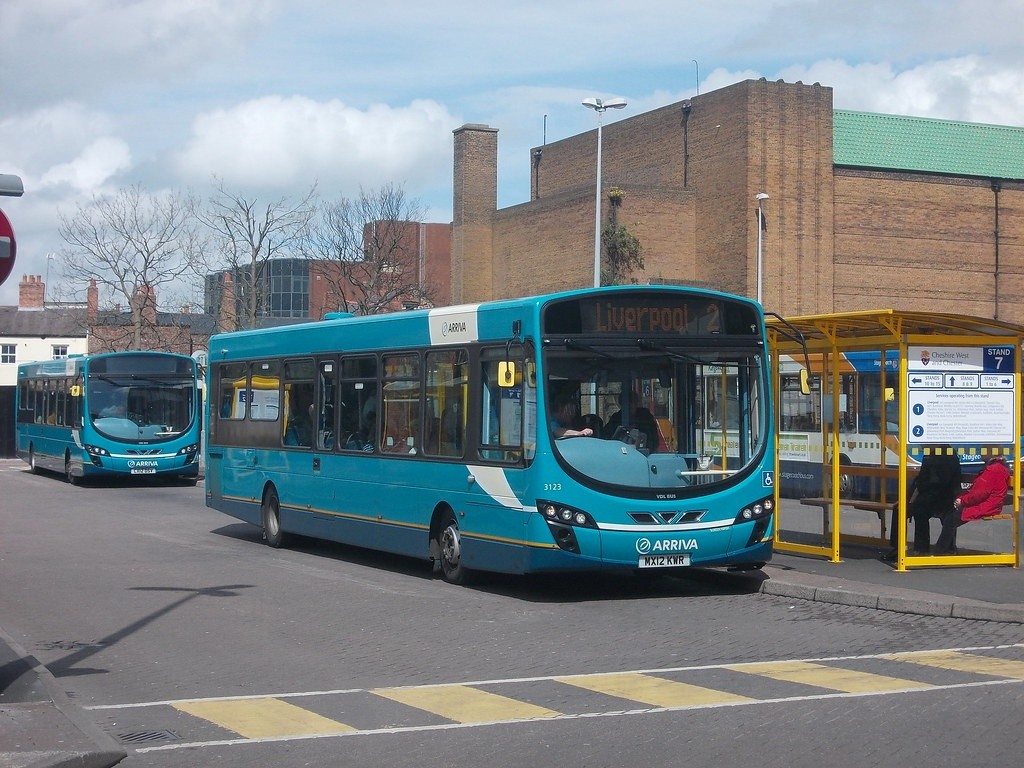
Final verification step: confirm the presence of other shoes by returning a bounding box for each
[882,549,898,564]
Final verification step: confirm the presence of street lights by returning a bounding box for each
[756,193,770,309]
[583,97,627,287]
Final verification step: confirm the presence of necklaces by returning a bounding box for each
[929,445,1011,556]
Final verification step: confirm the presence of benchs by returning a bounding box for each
[799,464,1014,554]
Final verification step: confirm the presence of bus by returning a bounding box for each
[642,351,990,506]
[16,352,202,486]
[203,285,777,588]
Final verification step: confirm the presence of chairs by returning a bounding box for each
[285,414,367,450]
[575,413,603,440]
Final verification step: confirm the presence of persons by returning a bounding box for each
[98,398,128,418]
[697,401,721,428]
[603,390,660,452]
[892,444,961,555]
[550,397,594,439]
[37,411,46,423]
[48,405,61,424]
